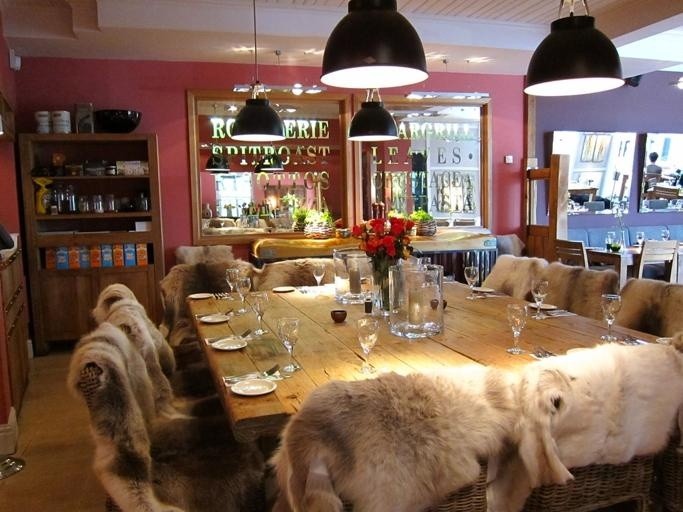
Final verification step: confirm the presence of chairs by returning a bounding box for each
[556,240,589,269]
[569,225,682,246]
[639,241,678,284]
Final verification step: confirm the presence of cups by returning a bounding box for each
[333,258,377,306]
[389,264,443,340]
[605,239,611,249]
[610,241,621,251]
[331,310,347,323]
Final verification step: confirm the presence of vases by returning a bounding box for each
[619,231,628,254]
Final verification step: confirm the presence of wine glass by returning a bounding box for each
[601,293,621,341]
[533,279,549,319]
[507,304,527,355]
[606,230,672,248]
[465,266,478,300]
[358,319,377,374]
[312,264,326,297]
[277,317,302,372]
[236,277,250,314]
[226,269,238,300]
[249,289,269,334]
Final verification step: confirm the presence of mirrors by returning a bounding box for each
[548,130,683,213]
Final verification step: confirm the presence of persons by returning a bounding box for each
[644,151,663,201]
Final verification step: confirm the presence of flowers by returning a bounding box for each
[353,217,415,311]
[612,204,626,249]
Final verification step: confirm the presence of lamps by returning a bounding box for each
[320,0,430,141]
[232,0,285,143]
[523,0,625,96]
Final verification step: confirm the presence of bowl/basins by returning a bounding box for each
[95,109,142,132]
[34,110,71,136]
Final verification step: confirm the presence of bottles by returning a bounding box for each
[34,178,151,214]
[260,200,269,213]
[202,203,213,217]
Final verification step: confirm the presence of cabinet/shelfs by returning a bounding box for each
[18,133,171,356]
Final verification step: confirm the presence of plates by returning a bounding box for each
[273,286,295,292]
[566,347,592,355]
[200,315,230,324]
[190,292,213,299]
[528,351,564,361]
[528,303,557,310]
[473,287,494,293]
[231,378,277,397]
[211,338,248,352]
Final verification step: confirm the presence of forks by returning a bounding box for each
[198,308,233,317]
[538,345,556,357]
[532,349,543,358]
[543,309,570,316]
[207,330,252,343]
[598,335,640,346]
[226,365,280,378]
